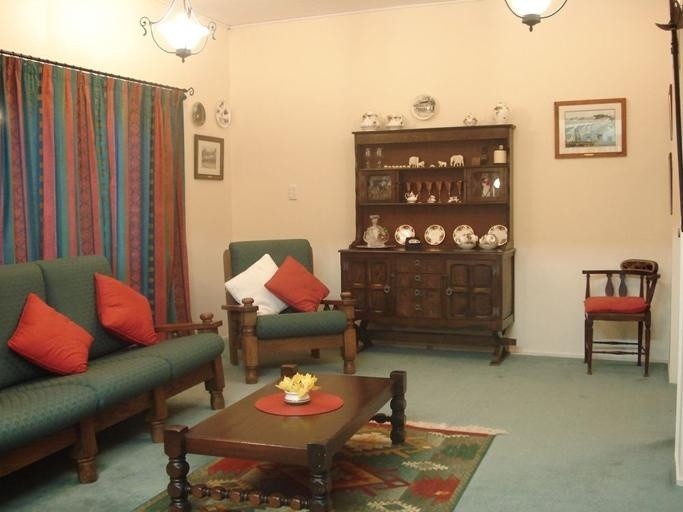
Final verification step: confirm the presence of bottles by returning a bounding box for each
[463,113,477,127]
[362,214,388,247]
[492,100,510,125]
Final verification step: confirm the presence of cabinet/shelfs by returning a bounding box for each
[336,246,518,364]
[352,125,517,249]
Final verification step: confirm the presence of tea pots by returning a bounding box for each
[359,111,381,130]
[454,231,476,250]
[385,112,404,130]
[479,234,498,249]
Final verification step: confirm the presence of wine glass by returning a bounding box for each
[403,180,462,204]
[360,146,382,169]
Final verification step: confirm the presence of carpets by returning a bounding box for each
[131,415,510,511]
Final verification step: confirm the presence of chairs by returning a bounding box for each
[218,238,363,386]
[577,257,662,379]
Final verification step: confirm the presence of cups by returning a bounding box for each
[283,391,311,404]
[492,150,506,163]
[404,237,421,250]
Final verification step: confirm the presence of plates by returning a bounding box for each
[411,94,436,121]
[355,245,395,250]
[488,225,507,246]
[423,225,444,246]
[453,224,473,244]
[214,97,231,129]
[191,101,205,127]
[394,223,414,246]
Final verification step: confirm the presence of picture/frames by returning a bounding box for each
[194,133,224,182]
[553,98,627,161]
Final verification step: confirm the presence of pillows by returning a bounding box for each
[222,254,291,316]
[6,288,94,374]
[89,268,157,349]
[263,252,331,315]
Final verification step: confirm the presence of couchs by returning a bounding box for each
[0,251,227,487]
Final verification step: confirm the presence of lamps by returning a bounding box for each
[135,0,224,65]
[499,1,571,35]
